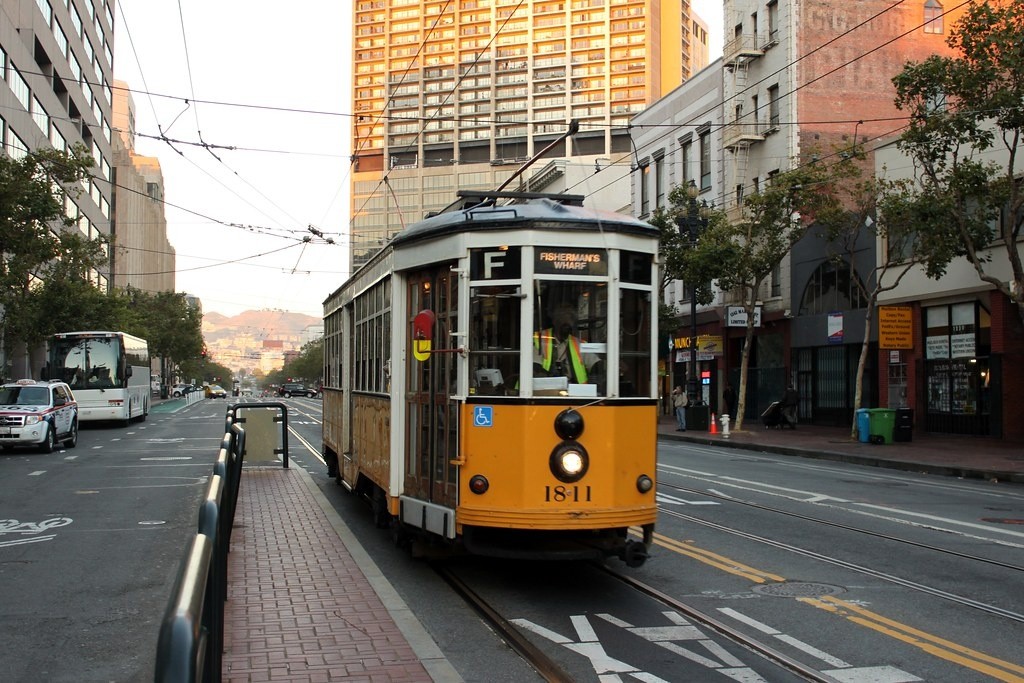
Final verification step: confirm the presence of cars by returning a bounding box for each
[203,384,226,399]
[168,383,195,398]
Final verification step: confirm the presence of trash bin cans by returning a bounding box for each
[856,407,869,442]
[686,400,708,431]
[867,408,896,445]
[894,408,913,443]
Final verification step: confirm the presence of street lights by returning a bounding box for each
[672,176,721,433]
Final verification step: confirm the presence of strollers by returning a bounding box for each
[763,388,798,431]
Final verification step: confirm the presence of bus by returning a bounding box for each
[40,332,151,427]
[151,374,161,394]
[241,389,253,397]
[319,189,660,573]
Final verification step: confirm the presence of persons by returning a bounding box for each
[723,384,738,419]
[782,383,802,428]
[670,385,689,433]
[512,303,630,396]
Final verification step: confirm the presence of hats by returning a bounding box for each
[89,376,98,382]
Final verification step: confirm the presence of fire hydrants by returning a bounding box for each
[720,413,731,439]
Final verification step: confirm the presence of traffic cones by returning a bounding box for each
[708,412,719,434]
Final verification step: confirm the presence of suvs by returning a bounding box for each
[279,384,318,398]
[0,378,79,453]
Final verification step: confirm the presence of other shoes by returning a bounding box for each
[679,429,685,432]
[676,429,679,431]
